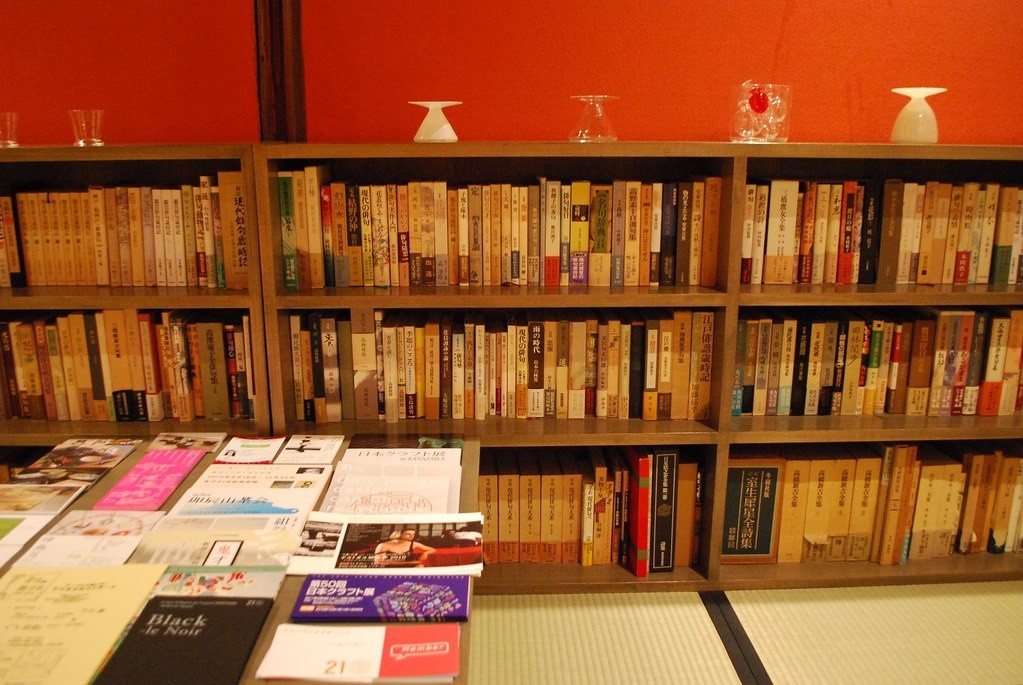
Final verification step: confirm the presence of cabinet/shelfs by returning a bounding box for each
[714,144,1023,592]
[0,143,271,447]
[271,141,741,596]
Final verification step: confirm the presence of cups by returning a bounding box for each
[0,111,21,147]
[729,80,792,143]
[69,109,106,148]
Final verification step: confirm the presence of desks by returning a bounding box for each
[0,435,481,685]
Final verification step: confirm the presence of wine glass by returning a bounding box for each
[568,94,619,142]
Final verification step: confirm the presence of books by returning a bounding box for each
[0,168,1023,685]
